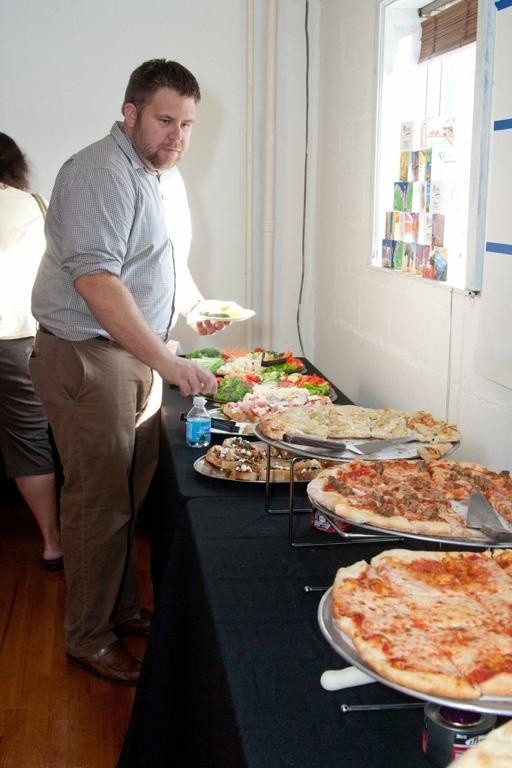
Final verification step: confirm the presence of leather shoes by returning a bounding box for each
[44,552,61,567]
[66,607,153,682]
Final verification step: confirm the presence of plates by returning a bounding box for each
[202,309,255,321]
[207,368,338,437]
[317,548,512,718]
[193,441,322,484]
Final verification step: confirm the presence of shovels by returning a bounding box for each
[465,488,512,543]
[283,433,416,455]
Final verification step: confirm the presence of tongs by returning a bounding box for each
[180,413,240,433]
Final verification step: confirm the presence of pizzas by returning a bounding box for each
[329,544,512,702]
[441,717,512,767]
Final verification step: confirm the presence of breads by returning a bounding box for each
[219,398,246,423]
[204,435,333,483]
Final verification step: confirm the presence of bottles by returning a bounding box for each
[185,396,211,448]
[423,703,496,767]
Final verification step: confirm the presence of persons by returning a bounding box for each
[1,131,64,568]
[26,56,235,684]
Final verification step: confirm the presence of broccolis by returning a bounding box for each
[213,375,252,404]
